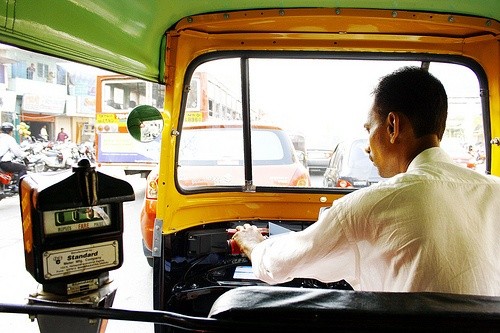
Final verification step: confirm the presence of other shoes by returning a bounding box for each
[13,186,19,192]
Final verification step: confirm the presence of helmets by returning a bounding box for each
[2,122,13,129]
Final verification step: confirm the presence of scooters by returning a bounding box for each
[0,139,95,199]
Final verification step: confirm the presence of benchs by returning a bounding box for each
[207,286,500,333]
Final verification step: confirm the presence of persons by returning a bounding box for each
[44,72,55,83]
[87,87,97,96]
[232,66,500,297]
[62,72,74,95]
[26,63,36,80]
[0,122,69,182]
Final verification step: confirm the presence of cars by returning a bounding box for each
[140,122,311,268]
[289,134,336,176]
[440,138,486,170]
[321,137,383,188]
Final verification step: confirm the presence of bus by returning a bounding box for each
[94,70,259,180]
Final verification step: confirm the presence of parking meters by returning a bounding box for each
[19,158,136,333]
